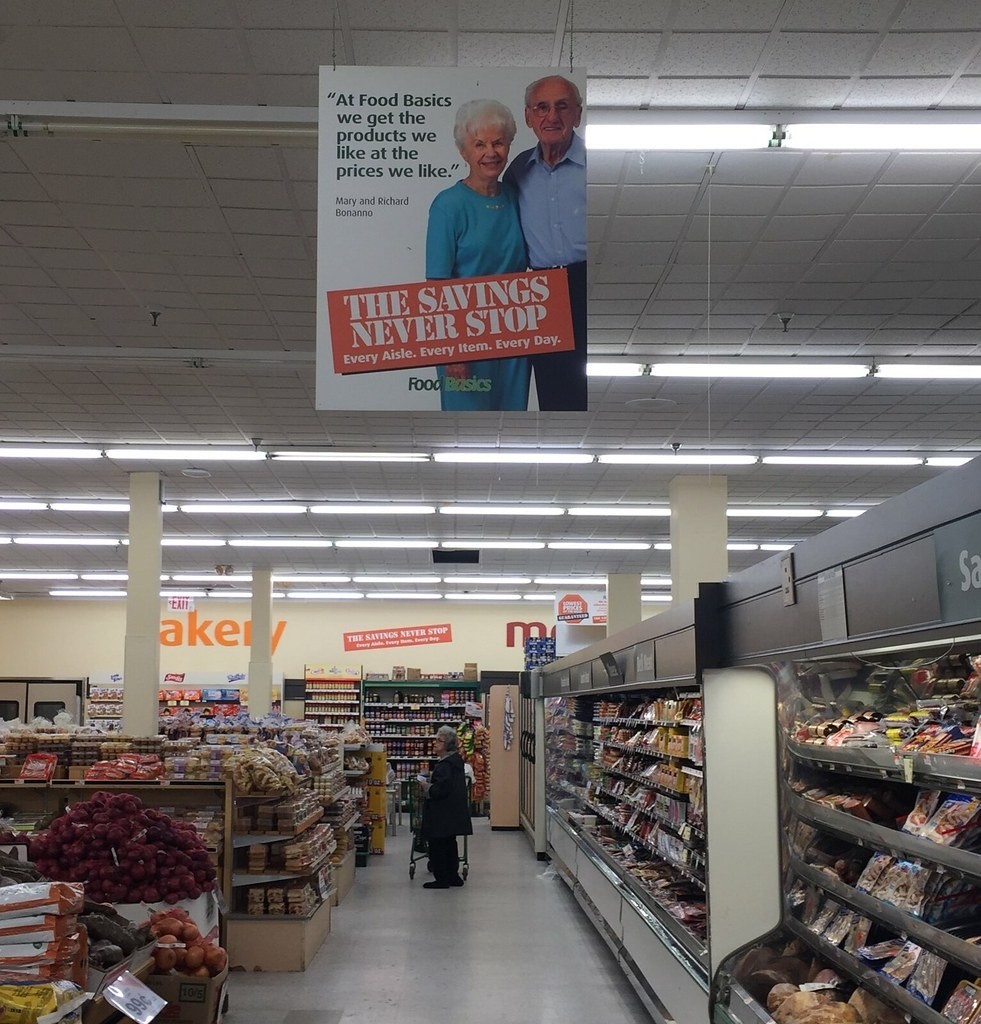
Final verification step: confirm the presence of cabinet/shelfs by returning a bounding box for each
[542,456,981,1024]
[303,677,362,734]
[0,739,370,973]
[364,682,480,817]
[84,683,281,726]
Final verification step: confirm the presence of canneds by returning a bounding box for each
[365,691,474,780]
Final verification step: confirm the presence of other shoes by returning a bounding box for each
[424,876,464,888]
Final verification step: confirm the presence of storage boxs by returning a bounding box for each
[525,636,563,670]
[465,663,478,681]
[364,752,387,855]
[0,765,66,779]
[86,939,229,1024]
[159,704,280,715]
[159,688,278,701]
[68,766,93,780]
[88,688,124,730]
[407,668,420,682]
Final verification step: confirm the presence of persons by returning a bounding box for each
[420,726,474,889]
[424,97,533,410]
[501,75,588,411]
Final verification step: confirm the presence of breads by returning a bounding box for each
[164,719,354,914]
[767,969,908,1024]
[0,726,168,766]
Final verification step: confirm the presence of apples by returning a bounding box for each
[0,791,217,904]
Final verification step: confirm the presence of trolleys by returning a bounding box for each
[407,774,472,881]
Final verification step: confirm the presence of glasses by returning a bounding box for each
[527,104,579,117]
[435,737,445,742]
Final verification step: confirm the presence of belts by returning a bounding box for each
[530,262,586,272]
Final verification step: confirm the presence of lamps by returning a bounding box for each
[0,442,981,467]
[586,109,981,151]
[0,346,981,378]
[0,534,794,550]
[0,498,868,518]
[0,100,319,140]
